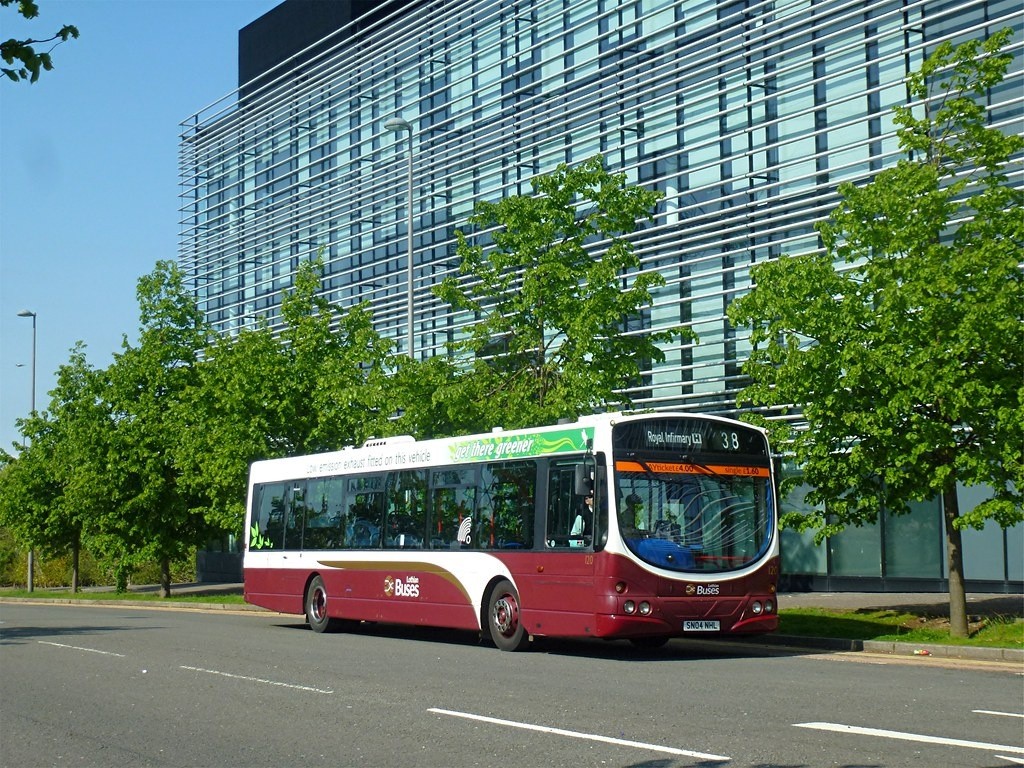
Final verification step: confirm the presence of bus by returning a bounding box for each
[242,411,780,654]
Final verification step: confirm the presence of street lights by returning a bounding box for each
[383,117,414,360]
[17,310,36,410]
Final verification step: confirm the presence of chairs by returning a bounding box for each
[329,509,528,550]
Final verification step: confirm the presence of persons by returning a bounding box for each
[570,495,594,535]
[620,493,644,530]
